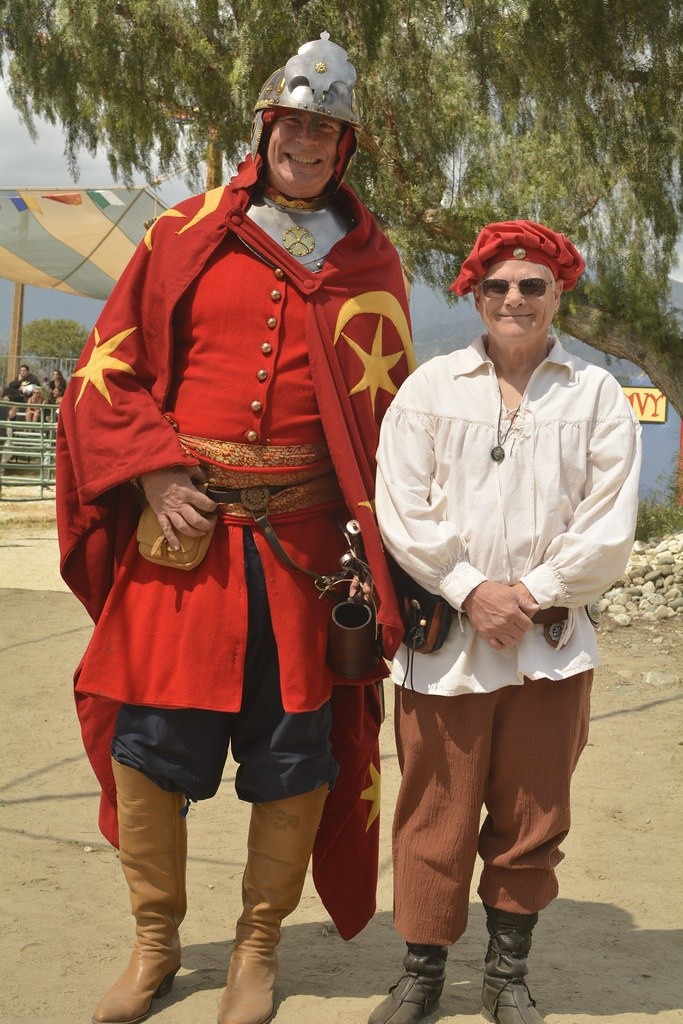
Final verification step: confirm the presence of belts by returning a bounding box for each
[193,480,294,506]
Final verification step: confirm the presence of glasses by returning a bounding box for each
[478,277,554,299]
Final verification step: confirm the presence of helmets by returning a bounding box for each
[251,32,365,164]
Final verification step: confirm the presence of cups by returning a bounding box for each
[329,587,381,679]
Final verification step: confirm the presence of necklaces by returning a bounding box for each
[491,387,521,461]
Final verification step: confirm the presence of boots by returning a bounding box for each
[92,756,192,1024]
[215,782,333,1024]
[477,901,549,1024]
[365,942,449,1024]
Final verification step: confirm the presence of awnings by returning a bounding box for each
[0,187,168,301]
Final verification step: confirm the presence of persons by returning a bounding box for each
[55,33,414,1024]
[0,365,67,424]
[365,219,644,1024]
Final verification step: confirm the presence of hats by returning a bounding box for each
[448,220,586,296]
